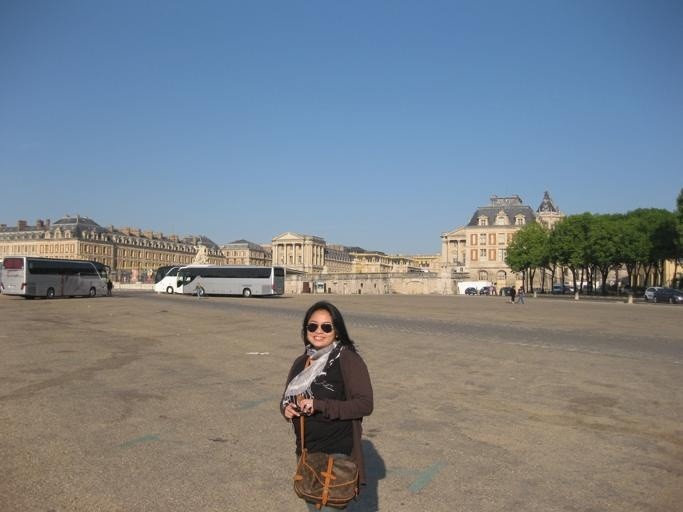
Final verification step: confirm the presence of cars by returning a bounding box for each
[526,281,589,294]
[499,287,517,296]
[480,286,491,296]
[465,286,478,295]
[653,288,683,303]
[645,287,666,301]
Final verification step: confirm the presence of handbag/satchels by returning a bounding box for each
[293,451,359,510]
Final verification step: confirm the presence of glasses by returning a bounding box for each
[305,323,333,333]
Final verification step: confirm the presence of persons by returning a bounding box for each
[280,301,373,512]
[509,286,516,304]
[105,279,114,296]
[518,286,525,304]
[193,282,202,300]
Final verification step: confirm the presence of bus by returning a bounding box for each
[175,265,285,296]
[37,258,111,283]
[153,265,185,295]
[0,255,108,299]
